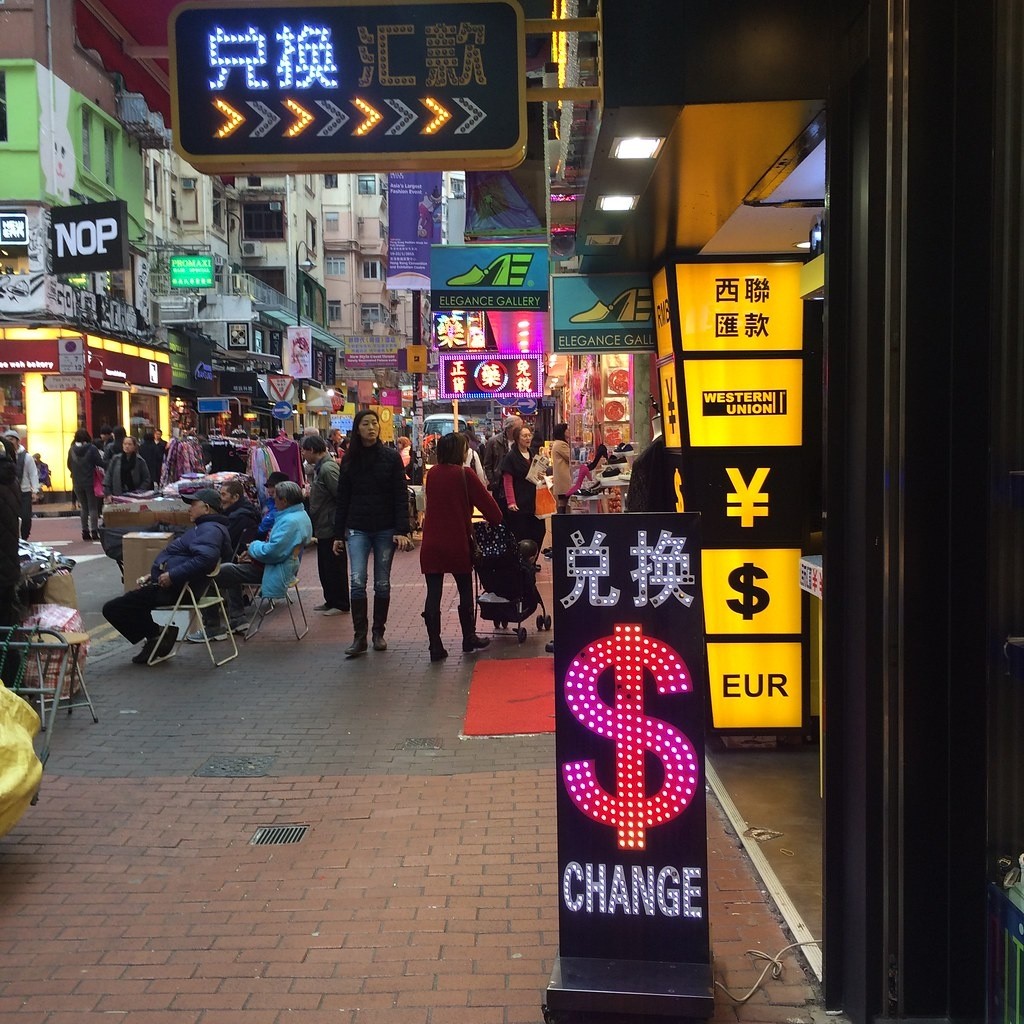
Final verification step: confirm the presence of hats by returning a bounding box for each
[32,453,41,461]
[182,488,223,513]
[4,429,21,440]
[264,471,290,488]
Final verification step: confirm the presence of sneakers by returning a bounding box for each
[225,616,249,634]
[186,626,228,643]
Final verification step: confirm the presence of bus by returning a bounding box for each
[423,413,472,443]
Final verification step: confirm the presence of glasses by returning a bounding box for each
[520,434,533,438]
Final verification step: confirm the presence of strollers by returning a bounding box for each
[470,511,552,644]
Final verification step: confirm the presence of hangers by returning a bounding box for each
[270,428,293,449]
[228,438,253,458]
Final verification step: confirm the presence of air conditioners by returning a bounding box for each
[269,202,282,212]
[359,217,364,224]
[181,177,197,191]
[242,240,264,259]
[392,312,399,321]
[362,322,373,332]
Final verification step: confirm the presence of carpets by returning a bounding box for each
[460,658,555,733]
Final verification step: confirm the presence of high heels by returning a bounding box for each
[575,482,603,495]
[566,465,592,497]
[588,444,608,471]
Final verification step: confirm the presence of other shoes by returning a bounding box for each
[601,442,634,478]
[82,530,100,541]
[223,594,250,607]
[314,604,351,616]
[133,625,179,664]
[70,504,80,511]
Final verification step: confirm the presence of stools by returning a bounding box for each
[29,633,99,731]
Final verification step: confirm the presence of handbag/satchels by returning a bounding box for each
[17,604,90,702]
[397,537,415,552]
[90,445,105,498]
[534,479,557,519]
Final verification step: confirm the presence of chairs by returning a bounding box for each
[241,538,318,642]
[147,558,238,668]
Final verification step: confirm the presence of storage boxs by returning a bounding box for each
[121,529,176,593]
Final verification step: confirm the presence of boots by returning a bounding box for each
[371,596,390,649]
[421,611,447,662]
[458,605,490,652]
[345,597,369,656]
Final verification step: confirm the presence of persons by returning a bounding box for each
[0,407,568,667]
[625,415,673,513]
